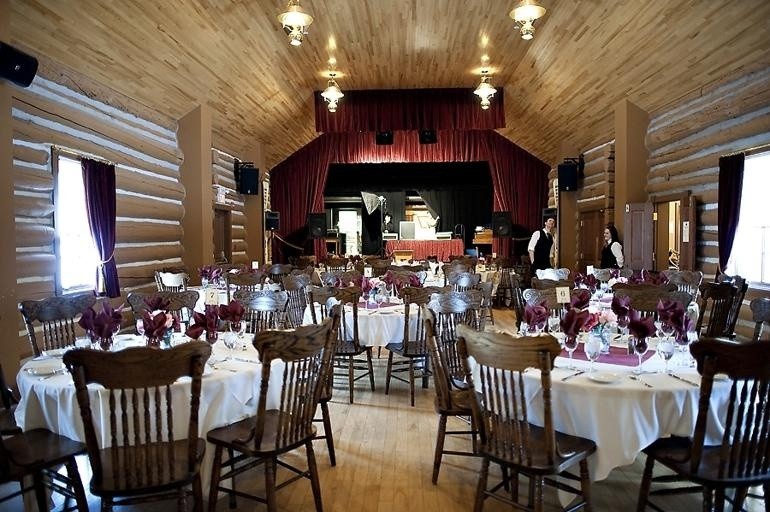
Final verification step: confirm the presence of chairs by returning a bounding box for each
[423,319,478,486]
[2,254,768,409]
[447,321,598,511]
[205,315,327,510]
[0,425,93,511]
[640,337,769,510]
[61,343,202,511]
[278,295,343,473]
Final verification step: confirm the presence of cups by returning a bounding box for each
[480,265,485,272]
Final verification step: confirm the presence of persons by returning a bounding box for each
[600,226,625,269]
[527,216,556,277]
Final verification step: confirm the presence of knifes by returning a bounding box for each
[561,371,584,382]
[39,373,56,381]
[630,376,652,388]
[368,311,378,315]
[668,374,699,388]
[235,356,262,365]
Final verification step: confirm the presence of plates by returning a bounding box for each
[380,310,394,314]
[587,372,620,383]
[28,363,66,377]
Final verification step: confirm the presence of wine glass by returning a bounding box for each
[596,284,608,305]
[519,314,680,375]
[200,274,226,292]
[70,316,249,374]
[360,292,385,312]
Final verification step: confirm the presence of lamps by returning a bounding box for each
[508,0,546,41]
[473,70,498,110]
[319,72,345,112]
[374,131,394,145]
[360,191,387,259]
[277,0,315,45]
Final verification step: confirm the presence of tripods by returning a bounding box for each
[375,202,392,259]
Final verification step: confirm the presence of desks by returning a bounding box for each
[473,238,492,258]
[12,328,317,504]
[314,237,340,266]
[386,238,465,263]
[465,331,769,511]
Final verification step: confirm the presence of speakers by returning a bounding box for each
[265,212,280,231]
[558,164,577,191]
[376,130,393,145]
[240,168,258,195]
[309,213,327,239]
[542,208,557,228]
[0,41,38,87]
[492,211,511,238]
